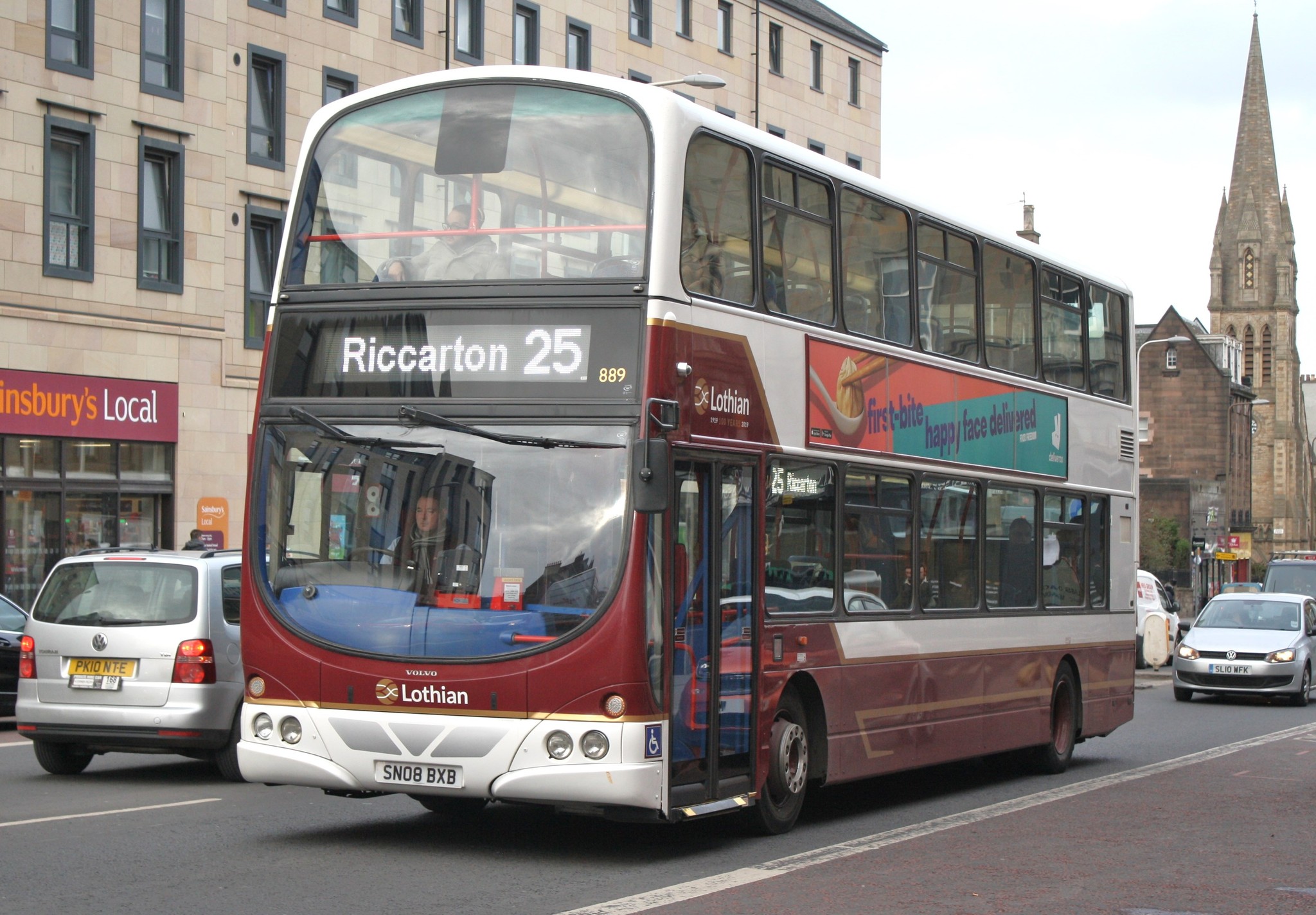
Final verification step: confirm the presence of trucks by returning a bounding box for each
[1258,549,1316,628]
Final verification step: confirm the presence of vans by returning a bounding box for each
[1220,581,1263,594]
[1135,569,1183,669]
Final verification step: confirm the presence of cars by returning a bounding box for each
[0,592,29,717]
[647,553,889,750]
[1172,592,1316,707]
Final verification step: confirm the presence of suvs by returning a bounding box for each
[15,547,320,782]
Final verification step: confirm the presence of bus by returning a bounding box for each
[238,65,1136,842]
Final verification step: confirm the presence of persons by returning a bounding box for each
[182,529,208,551]
[890,565,931,609]
[380,491,472,591]
[1164,579,1177,604]
[681,200,724,296]
[1002,518,1036,608]
[385,204,502,282]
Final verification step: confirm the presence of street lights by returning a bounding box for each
[1134,335,1193,568]
[1224,398,1270,583]
